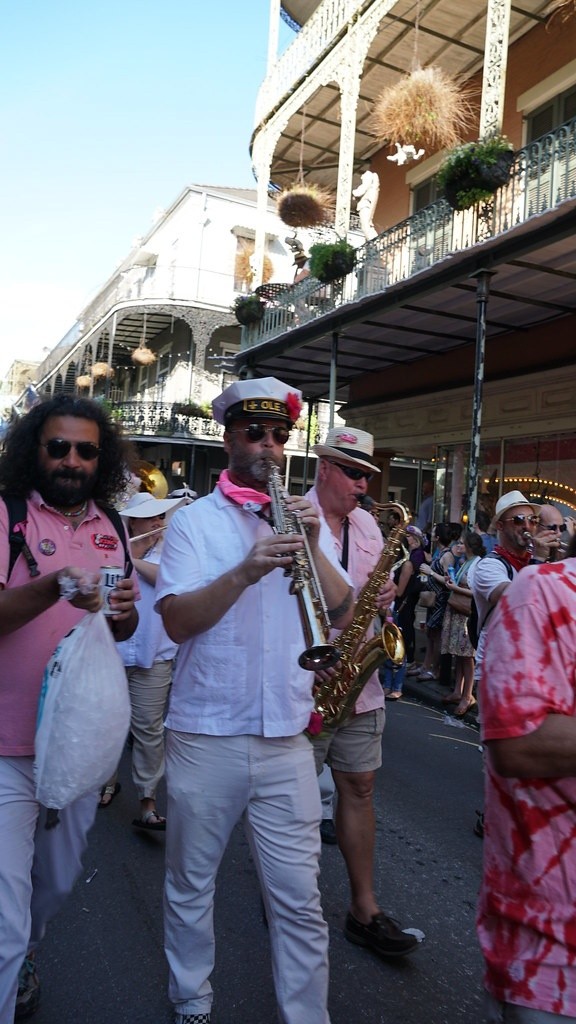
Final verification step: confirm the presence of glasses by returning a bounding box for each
[226,425,292,444]
[328,460,373,482]
[137,512,167,522]
[538,522,567,534]
[38,437,104,461]
[501,514,539,526]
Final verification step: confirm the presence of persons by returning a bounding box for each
[292,252,311,282]
[153,375,355,1024]
[361,490,567,714]
[475,556,576,1024]
[97,492,186,831]
[304,426,417,956]
[352,171,379,242]
[0,391,142,1024]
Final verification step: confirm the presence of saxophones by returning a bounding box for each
[312,492,415,730]
[265,458,344,672]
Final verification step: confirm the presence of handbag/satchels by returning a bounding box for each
[417,590,436,609]
[447,590,472,618]
[32,574,134,810]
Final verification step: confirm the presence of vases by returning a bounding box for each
[234,301,265,326]
[443,151,514,212]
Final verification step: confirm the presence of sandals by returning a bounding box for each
[455,697,478,715]
[442,693,464,701]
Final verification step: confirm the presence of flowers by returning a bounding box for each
[229,294,260,311]
[286,393,302,422]
[433,132,514,193]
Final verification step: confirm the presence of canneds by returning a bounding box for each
[98,566,125,617]
[421,571,428,582]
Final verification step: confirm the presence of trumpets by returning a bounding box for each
[522,531,570,564]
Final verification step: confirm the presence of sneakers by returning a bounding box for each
[342,911,418,957]
[13,949,41,1014]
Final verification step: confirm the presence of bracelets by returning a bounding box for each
[430,572,435,576]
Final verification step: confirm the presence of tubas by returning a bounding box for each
[128,459,170,500]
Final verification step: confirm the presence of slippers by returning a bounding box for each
[98,782,121,809]
[130,811,168,829]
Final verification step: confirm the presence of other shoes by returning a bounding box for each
[415,671,440,680]
[320,817,337,844]
[474,808,485,838]
[407,666,427,676]
[384,691,404,701]
[173,1011,211,1024]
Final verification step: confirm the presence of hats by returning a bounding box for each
[212,377,304,424]
[117,493,185,527]
[487,490,541,536]
[312,427,382,473]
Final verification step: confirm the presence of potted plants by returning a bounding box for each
[308,243,358,283]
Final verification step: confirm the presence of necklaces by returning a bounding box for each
[63,501,87,516]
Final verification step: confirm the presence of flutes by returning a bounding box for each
[128,525,168,543]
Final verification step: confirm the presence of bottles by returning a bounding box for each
[447,564,456,582]
[421,563,428,582]
